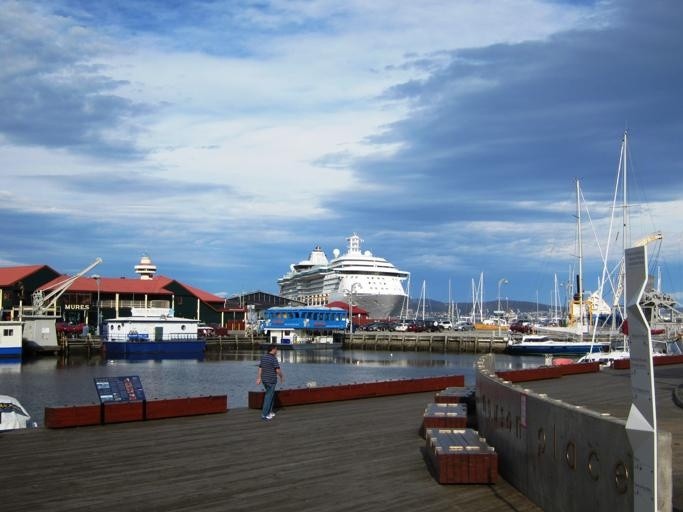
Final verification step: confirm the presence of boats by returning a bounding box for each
[197,323,213,335]
[0,394,35,429]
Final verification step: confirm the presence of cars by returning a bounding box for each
[362,318,476,332]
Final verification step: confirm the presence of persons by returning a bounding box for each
[257,344,282,419]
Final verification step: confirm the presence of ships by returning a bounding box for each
[271,230,410,319]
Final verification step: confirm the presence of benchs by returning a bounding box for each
[434,386,475,403]
[247,376,464,409]
[102,400,144,424]
[496,354,683,382]
[424,403,468,428]
[427,428,498,485]
[44,403,103,430]
[146,394,227,428]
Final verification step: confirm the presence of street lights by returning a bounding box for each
[91,273,100,335]
